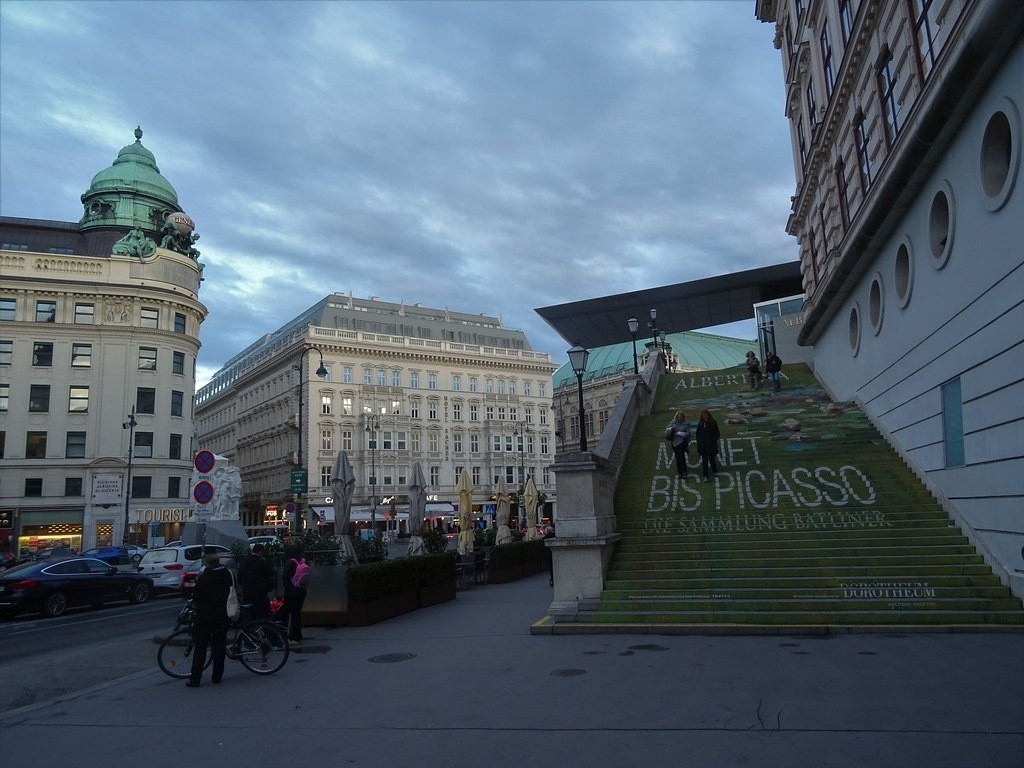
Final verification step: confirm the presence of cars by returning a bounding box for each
[128,544,148,562]
[0,547,77,571]
[181,554,235,595]
[248,537,281,549]
[522,524,547,541]
[137,544,231,598]
[0,556,153,617]
[79,547,128,565]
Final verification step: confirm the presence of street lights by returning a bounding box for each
[292,347,328,544]
[365,415,380,529]
[650,308,658,347]
[122,414,137,544]
[665,344,672,371]
[660,331,666,353]
[513,420,530,487]
[627,317,639,374]
[567,341,590,452]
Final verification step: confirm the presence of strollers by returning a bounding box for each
[245,598,290,649]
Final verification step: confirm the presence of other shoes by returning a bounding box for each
[273,646,284,651]
[186,680,196,686]
[288,640,299,646]
[713,472,717,477]
[703,476,708,483]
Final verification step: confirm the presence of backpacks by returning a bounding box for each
[290,559,311,589]
[768,356,780,372]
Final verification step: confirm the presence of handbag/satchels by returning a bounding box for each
[226,568,240,623]
[666,420,678,441]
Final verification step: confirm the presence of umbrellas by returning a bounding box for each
[407,462,427,557]
[524,479,538,542]
[456,468,475,555]
[328,450,359,567]
[496,475,512,545]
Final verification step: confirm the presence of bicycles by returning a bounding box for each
[156,598,289,678]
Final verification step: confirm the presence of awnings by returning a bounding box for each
[311,501,454,523]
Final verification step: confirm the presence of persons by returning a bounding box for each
[746,351,760,391]
[695,410,720,483]
[664,410,691,479]
[765,352,782,392]
[236,545,284,651]
[273,545,306,651]
[541,525,555,587]
[185,553,237,688]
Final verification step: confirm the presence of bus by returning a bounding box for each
[243,526,291,539]
